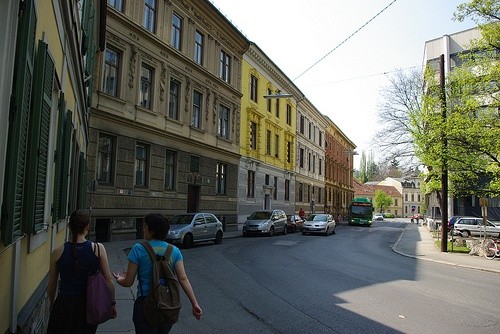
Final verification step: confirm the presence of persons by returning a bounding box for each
[47,209,117,334]
[111,213,202,334]
[299,208,305,220]
[411,214,419,225]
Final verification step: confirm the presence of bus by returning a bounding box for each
[347,197,375,227]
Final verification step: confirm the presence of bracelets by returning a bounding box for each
[112,302,116,305]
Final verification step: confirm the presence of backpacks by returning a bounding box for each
[139,241,181,326]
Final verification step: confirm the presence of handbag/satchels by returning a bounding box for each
[86,242,112,326]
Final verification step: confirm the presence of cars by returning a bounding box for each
[447,216,464,236]
[411,214,423,219]
[301,213,336,236]
[285,214,304,233]
[242,210,287,237]
[373,213,384,220]
[163,213,224,249]
[453,217,500,238]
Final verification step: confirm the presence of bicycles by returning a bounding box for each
[482,238,500,261]
